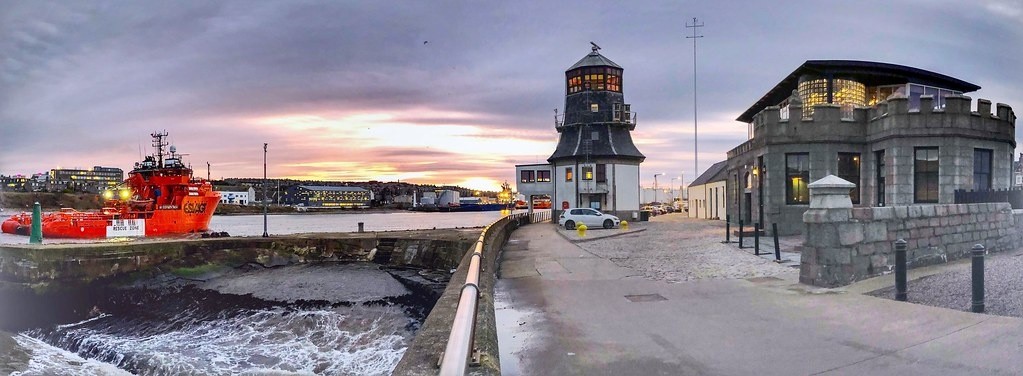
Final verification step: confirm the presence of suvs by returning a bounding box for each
[559,208,620,230]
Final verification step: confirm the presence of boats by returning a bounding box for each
[1,129,223,239]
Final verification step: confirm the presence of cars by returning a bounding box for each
[641,200,688,216]
[533,201,542,206]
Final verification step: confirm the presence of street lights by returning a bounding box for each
[685,17,705,183]
[672,177,680,202]
[654,173,666,204]
[262,142,269,238]
